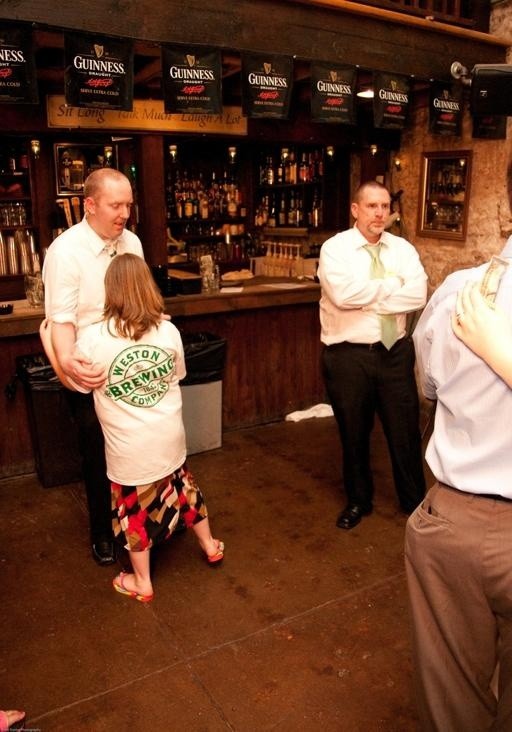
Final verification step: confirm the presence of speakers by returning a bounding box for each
[468,64,512,117]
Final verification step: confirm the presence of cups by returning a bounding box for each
[0,202,27,227]
[0,228,42,275]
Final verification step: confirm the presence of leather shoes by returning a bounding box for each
[92,539,117,564]
[337,502,375,529]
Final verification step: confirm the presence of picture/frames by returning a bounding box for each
[52,143,118,195]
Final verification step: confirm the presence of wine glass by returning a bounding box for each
[259,240,303,279]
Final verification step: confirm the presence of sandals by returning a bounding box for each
[0,708,26,732]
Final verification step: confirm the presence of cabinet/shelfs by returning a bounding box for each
[257,143,350,232]
[163,163,256,266]
[0,168,40,302]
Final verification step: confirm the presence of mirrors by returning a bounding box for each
[413,150,473,241]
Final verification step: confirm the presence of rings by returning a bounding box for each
[456,311,464,324]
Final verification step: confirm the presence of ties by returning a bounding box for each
[365,243,398,352]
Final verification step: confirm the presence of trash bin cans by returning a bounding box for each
[15,351,78,487]
[179,330,228,457]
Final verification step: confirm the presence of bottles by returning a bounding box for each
[255,148,325,232]
[305,243,322,258]
[59,146,117,194]
[425,162,465,232]
[163,168,259,263]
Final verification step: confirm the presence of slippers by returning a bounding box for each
[112,572,153,601]
[206,537,226,562]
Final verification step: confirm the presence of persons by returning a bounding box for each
[451,280,512,390]
[0,709,26,732]
[317,181,429,530]
[39,253,225,604]
[41,168,187,566]
[404,163,512,731]
